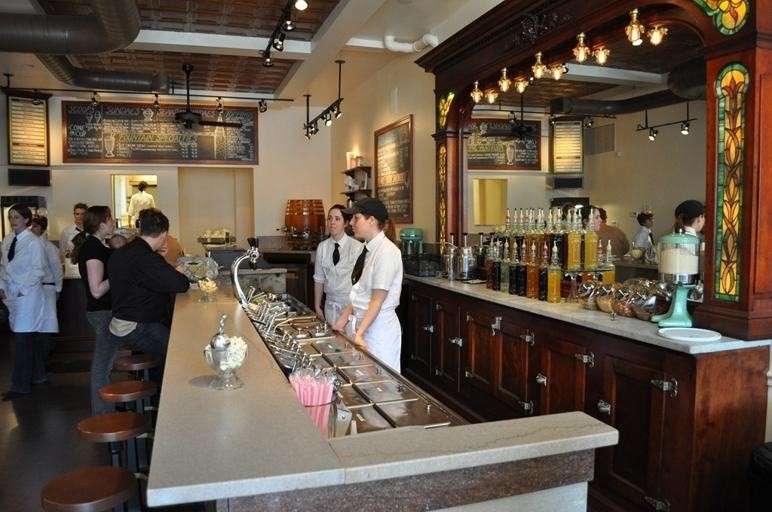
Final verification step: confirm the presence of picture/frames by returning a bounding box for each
[374,113,414,224]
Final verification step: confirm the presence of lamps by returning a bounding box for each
[484,24,668,104]
[303,59,346,140]
[633,100,699,141]
[31,88,295,113]
[259,0,309,67]
[470,6,645,104]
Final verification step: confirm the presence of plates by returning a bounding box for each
[657,327,722,342]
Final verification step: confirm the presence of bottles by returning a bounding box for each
[346,150,363,169]
[484,207,616,304]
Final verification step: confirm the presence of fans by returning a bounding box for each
[148,62,244,130]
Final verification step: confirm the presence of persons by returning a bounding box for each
[312,204,362,338]
[78,206,117,468]
[655,200,705,264]
[631,211,657,264]
[129,181,155,227]
[0,204,48,395]
[561,203,629,259]
[330,198,407,374]
[351,355,407,430]
[107,208,189,377]
[29,203,184,334]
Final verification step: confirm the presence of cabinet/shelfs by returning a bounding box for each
[339,166,372,205]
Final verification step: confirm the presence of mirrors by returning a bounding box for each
[458,22,707,303]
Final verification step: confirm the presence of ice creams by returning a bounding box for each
[204,314,248,370]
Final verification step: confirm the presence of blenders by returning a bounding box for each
[650,224,701,327]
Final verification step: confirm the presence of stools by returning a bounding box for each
[40,347,166,511]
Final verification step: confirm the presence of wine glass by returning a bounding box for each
[198,280,222,303]
[203,342,248,391]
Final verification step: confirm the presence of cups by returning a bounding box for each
[305,391,339,439]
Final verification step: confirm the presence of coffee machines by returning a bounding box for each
[397,226,424,255]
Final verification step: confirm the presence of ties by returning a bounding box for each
[8,237,17,262]
[333,243,339,265]
[352,246,367,285]
[649,233,655,246]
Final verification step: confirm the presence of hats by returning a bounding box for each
[676,200,704,219]
[341,198,388,220]
